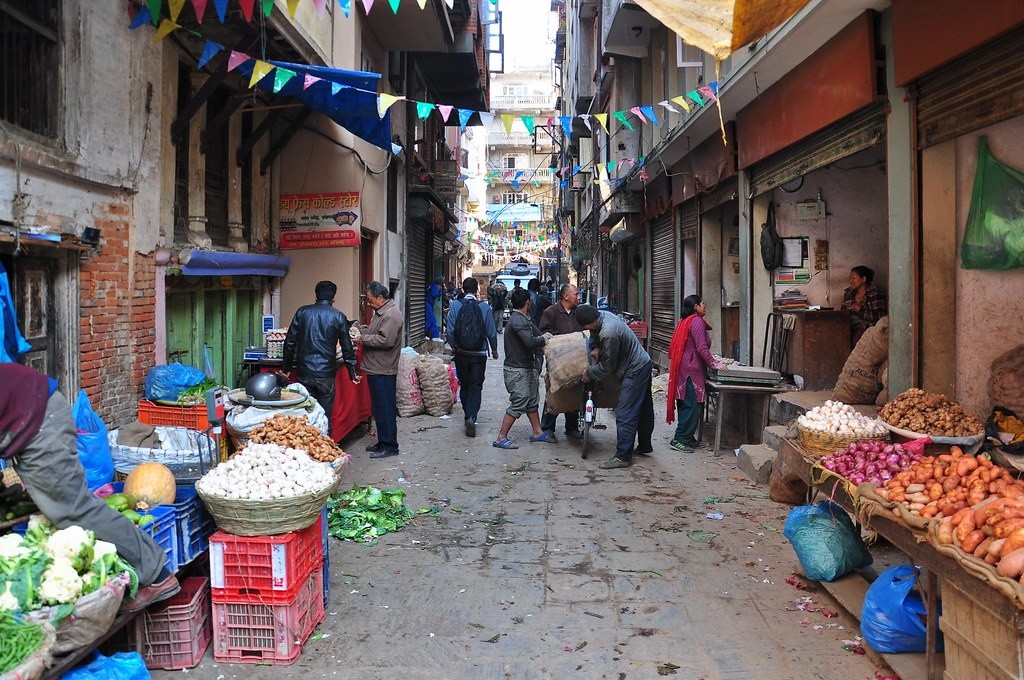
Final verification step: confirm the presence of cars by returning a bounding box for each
[494,261,541,326]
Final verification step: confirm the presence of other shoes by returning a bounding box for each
[499,330,504,334]
[565,430,582,439]
[634,445,642,454]
[544,429,554,436]
[598,456,631,469]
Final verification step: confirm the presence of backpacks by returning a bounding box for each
[454,297,491,357]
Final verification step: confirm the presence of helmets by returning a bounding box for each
[246,372,280,401]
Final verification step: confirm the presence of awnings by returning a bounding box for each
[170,61,403,176]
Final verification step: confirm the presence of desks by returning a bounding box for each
[697,378,788,457]
[721,305,740,358]
[773,308,851,391]
[261,345,373,447]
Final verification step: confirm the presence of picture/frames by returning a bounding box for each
[781,236,803,269]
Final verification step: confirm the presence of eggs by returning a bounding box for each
[271,326,288,339]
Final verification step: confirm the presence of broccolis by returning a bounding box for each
[0,514,117,610]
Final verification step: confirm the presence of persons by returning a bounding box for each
[493,284,655,469]
[447,277,499,437]
[669,294,729,453]
[487,276,555,386]
[0,362,182,614]
[841,266,886,349]
[424,276,466,338]
[349,281,404,458]
[279,280,363,438]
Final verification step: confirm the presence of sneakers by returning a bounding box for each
[669,439,694,453]
[689,438,698,446]
[465,417,476,437]
[425,336,430,341]
[432,337,444,342]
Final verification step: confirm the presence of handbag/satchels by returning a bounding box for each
[146,362,204,402]
[861,565,945,653]
[784,500,873,581]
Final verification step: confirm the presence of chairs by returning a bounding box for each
[493,195,500,204]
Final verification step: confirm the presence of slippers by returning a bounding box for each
[493,437,520,450]
[530,432,556,442]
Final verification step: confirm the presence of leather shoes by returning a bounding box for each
[370,449,399,459]
[119,573,180,612]
[365,441,382,451]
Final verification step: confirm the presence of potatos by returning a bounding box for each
[886,445,1024,585]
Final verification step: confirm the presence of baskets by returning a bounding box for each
[196,474,343,536]
[796,422,889,456]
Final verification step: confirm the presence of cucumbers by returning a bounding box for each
[0,472,39,520]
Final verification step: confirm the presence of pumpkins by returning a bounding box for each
[123,461,176,510]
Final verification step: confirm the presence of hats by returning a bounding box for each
[576,305,600,326]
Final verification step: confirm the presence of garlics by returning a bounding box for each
[797,400,887,433]
[199,441,333,500]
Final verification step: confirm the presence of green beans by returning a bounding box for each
[0,608,44,676]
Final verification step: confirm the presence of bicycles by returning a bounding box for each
[574,377,607,460]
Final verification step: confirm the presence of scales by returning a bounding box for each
[207,387,224,466]
[244,314,275,360]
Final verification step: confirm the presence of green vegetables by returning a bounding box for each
[326,481,431,546]
[175,377,218,402]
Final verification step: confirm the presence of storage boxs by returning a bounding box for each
[628,323,648,339]
[138,399,229,467]
[12,480,329,671]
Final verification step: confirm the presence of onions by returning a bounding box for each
[821,439,922,487]
